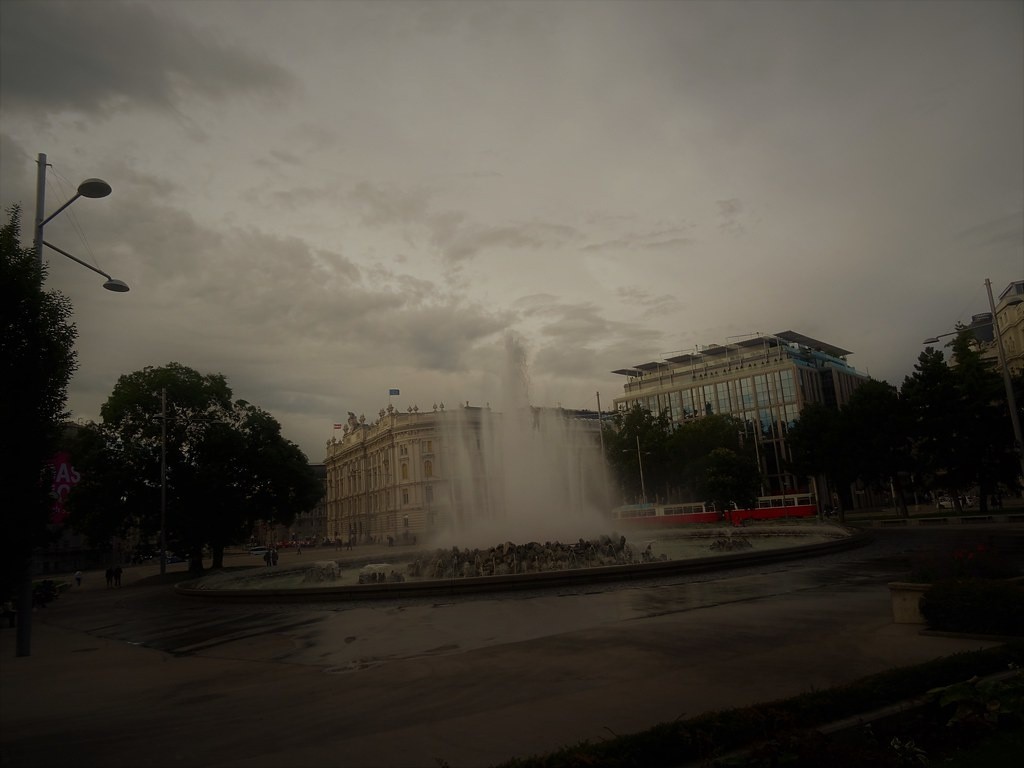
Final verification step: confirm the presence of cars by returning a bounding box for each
[936,495,980,509]
[249,546,277,558]
[139,550,189,564]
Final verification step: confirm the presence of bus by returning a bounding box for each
[611,493,822,528]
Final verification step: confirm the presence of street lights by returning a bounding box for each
[921,277,1024,480]
[152,387,224,589]
[26,150,130,314]
[622,436,652,503]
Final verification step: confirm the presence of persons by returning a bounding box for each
[105,564,124,588]
[321,537,353,551]
[388,536,394,547]
[263,547,279,568]
[0,598,18,628]
[74,569,84,588]
[990,494,1004,512]
[823,503,837,519]
[295,542,301,556]
